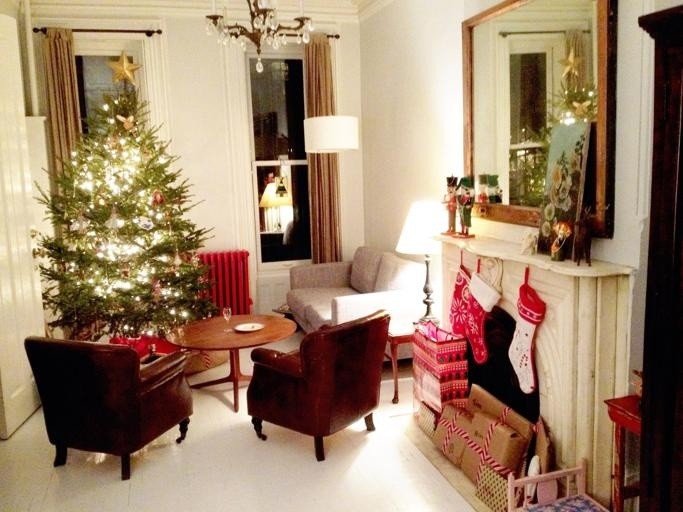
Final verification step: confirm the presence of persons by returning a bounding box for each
[454,177,475,237]
[441,177,458,235]
[488,175,503,203]
[478,175,488,203]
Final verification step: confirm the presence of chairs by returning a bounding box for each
[252,309,400,462]
[22,321,195,481]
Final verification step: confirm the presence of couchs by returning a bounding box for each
[287,241,429,338]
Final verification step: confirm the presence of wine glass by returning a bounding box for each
[222,307,232,333]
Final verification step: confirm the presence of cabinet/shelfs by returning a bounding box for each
[508,457,615,512]
[638,5,683,512]
[605,394,641,512]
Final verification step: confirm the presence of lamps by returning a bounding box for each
[260,181,292,234]
[395,197,451,323]
[206,0,316,75]
[305,40,360,153]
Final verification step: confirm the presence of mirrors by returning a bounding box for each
[462,0,614,239]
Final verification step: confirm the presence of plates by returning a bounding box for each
[235,323,263,332]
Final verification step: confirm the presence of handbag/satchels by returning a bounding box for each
[411,319,470,415]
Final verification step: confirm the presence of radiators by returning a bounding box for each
[192,251,254,315]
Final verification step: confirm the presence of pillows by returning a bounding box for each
[349,245,380,294]
[379,250,415,292]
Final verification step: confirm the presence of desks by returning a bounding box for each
[164,313,297,415]
